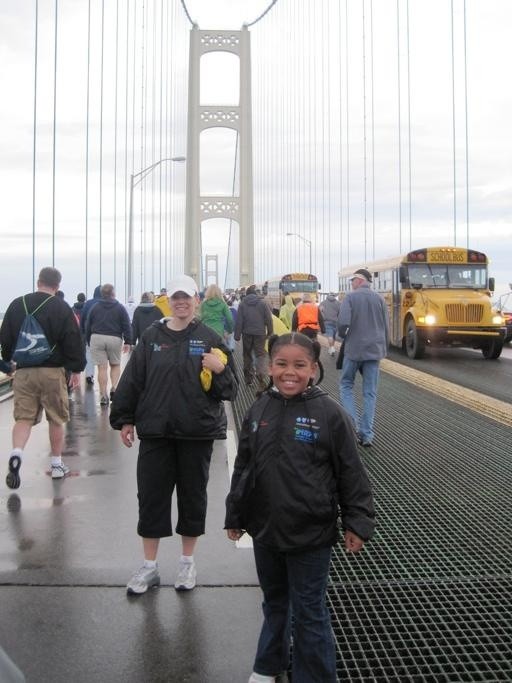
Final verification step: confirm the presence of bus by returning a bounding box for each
[263,273,323,319]
[335,246,501,361]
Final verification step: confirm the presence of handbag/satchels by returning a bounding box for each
[336,334,344,369]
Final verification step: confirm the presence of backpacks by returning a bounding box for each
[10,294,58,368]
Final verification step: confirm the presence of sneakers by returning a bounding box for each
[50,462,70,479]
[354,431,374,446]
[247,671,277,683]
[174,559,197,592]
[245,374,269,387]
[109,387,117,401]
[5,451,22,490]
[327,346,336,356]
[124,565,161,595]
[100,394,110,405]
[85,375,94,384]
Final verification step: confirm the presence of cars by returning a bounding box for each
[492,282,512,341]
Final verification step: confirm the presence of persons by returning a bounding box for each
[0,266,87,485]
[56,281,342,407]
[226,331,376,683]
[108,273,239,594]
[336,267,388,447]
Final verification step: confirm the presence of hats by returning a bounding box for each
[167,272,199,297]
[302,293,312,302]
[347,268,373,282]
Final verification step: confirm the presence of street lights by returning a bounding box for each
[123,156,187,303]
[286,232,313,273]
